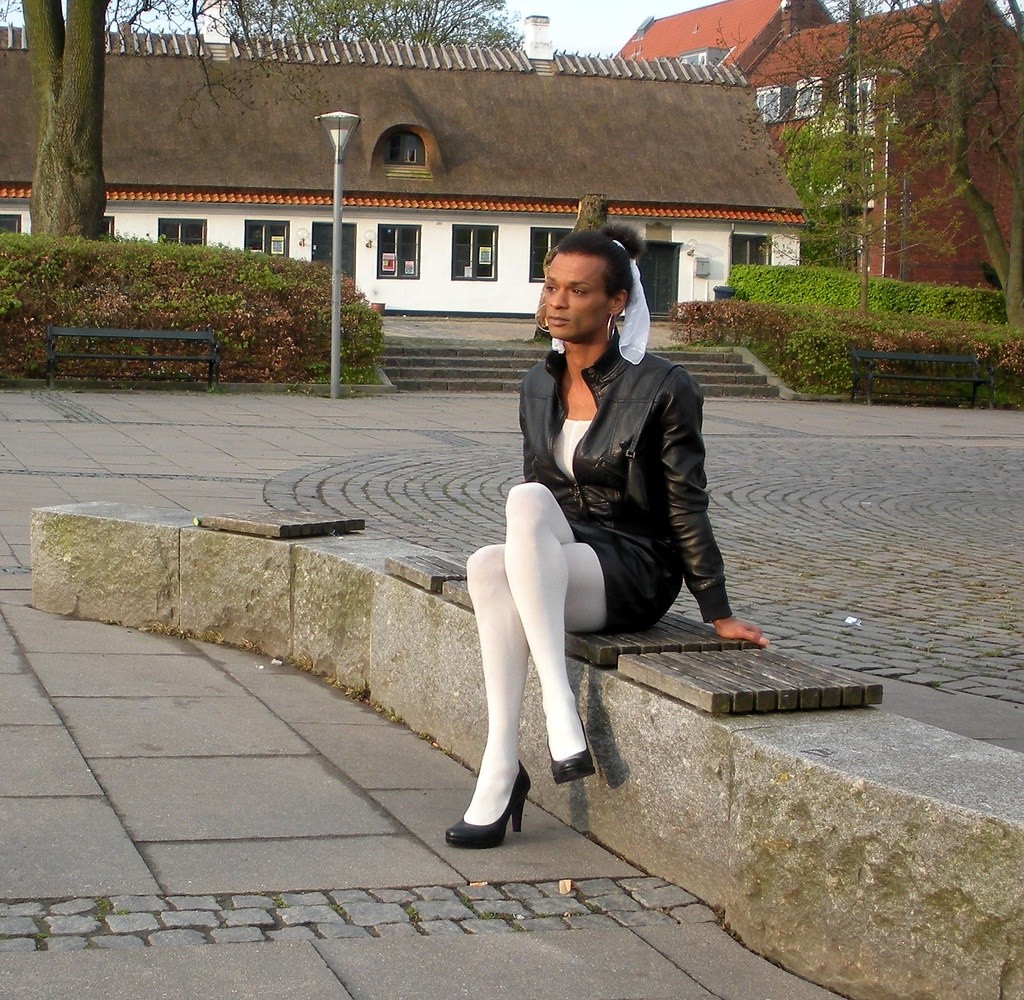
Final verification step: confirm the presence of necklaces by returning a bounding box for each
[445,224,768,847]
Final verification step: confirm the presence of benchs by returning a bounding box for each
[46,322,221,393]
[849,348,995,410]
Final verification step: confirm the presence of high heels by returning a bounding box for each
[445,759,531,849]
[546,710,596,785]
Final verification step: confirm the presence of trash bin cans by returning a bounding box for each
[713,285,737,300]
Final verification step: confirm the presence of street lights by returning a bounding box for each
[312,112,361,398]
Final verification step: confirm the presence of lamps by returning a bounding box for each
[686,239,697,257]
[365,229,376,248]
[296,228,310,247]
